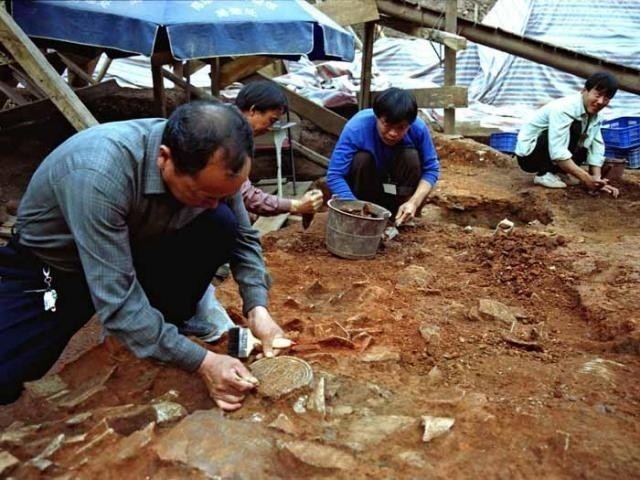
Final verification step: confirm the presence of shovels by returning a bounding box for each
[383,217,401,240]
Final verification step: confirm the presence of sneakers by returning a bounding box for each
[400,217,423,227]
[176,319,218,339]
[533,173,580,189]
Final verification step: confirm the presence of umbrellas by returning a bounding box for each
[5,0,358,71]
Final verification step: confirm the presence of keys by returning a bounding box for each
[23,265,58,313]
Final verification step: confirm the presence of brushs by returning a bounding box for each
[228,328,298,359]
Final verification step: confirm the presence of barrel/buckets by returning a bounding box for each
[325,199,392,259]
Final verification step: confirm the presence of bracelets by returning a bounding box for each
[293,199,299,213]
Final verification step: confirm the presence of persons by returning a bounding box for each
[512,72,622,200]
[1,101,295,414]
[220,81,325,218]
[325,87,442,229]
